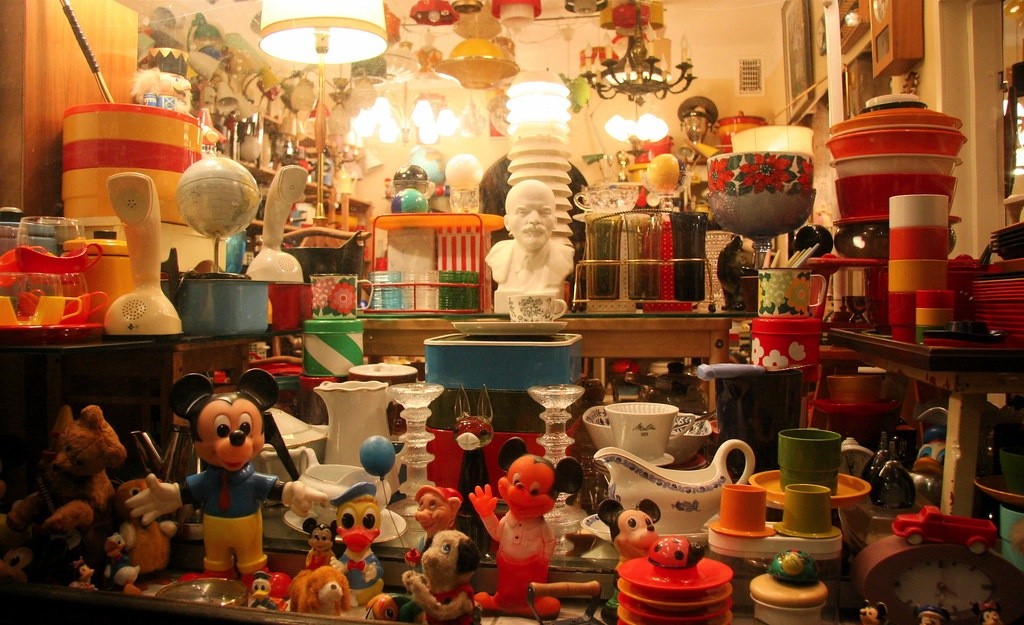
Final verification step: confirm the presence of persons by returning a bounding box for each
[485,179,576,289]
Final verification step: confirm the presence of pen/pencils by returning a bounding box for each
[763,243,821,269]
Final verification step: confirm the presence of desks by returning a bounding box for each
[45,338,259,452]
[828,328,1024,519]
[364,317,733,415]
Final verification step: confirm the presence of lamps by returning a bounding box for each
[134,0,817,275]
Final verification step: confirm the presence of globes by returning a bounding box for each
[176,158,261,278]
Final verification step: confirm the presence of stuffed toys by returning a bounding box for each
[6,403,128,538]
[114,476,177,577]
[289,565,352,616]
[401,529,483,625]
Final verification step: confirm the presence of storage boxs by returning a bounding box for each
[423,333,583,390]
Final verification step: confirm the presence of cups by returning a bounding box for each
[573,183,640,213]
[143,93,157,107]
[154,576,249,607]
[606,192,1024,625]
[347,364,418,442]
[506,294,567,323]
[258,447,317,508]
[450,185,479,214]
[368,269,479,309]
[0,216,108,325]
[157,94,177,110]
[309,274,373,320]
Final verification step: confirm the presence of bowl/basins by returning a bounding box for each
[824,94,969,218]
[582,405,712,466]
[384,181,435,202]
[268,282,312,334]
[161,278,270,334]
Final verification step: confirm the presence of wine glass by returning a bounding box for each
[706,151,817,269]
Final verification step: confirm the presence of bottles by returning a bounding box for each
[200,107,369,275]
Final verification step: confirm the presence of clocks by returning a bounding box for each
[849,531,1024,625]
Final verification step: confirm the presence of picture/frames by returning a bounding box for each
[781,0,816,125]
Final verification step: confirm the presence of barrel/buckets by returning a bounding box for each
[61,105,204,226]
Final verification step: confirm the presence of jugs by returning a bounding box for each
[131,425,206,542]
[314,380,411,504]
[592,439,756,534]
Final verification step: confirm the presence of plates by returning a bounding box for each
[284,509,407,544]
[580,515,710,548]
[452,321,566,336]
